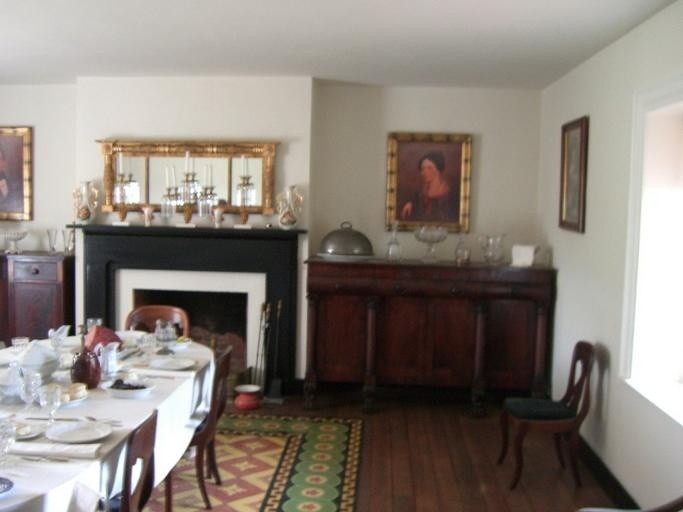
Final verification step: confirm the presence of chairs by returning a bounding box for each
[122,410,158,512]
[166,346,233,512]
[125,305,190,338]
[498,341,596,491]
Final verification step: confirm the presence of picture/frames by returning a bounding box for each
[385,130,474,232]
[0,125,33,221]
[558,112,589,233]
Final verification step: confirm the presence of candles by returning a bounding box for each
[119,152,124,175]
[241,155,245,176]
[185,151,189,174]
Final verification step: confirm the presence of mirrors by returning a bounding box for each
[96,140,282,214]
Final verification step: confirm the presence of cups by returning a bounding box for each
[478,232,506,264]
[62,229,73,256]
[47,229,57,255]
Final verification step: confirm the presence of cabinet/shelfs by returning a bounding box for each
[0,249,73,347]
[304,254,556,414]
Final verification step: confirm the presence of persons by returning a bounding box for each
[400,150,458,221]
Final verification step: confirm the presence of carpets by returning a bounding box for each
[142,413,364,512]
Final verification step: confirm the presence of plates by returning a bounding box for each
[316,252,376,262]
[0,331,197,496]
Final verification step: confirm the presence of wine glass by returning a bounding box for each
[386,219,401,262]
[0,318,178,465]
[455,227,470,264]
[414,224,449,264]
